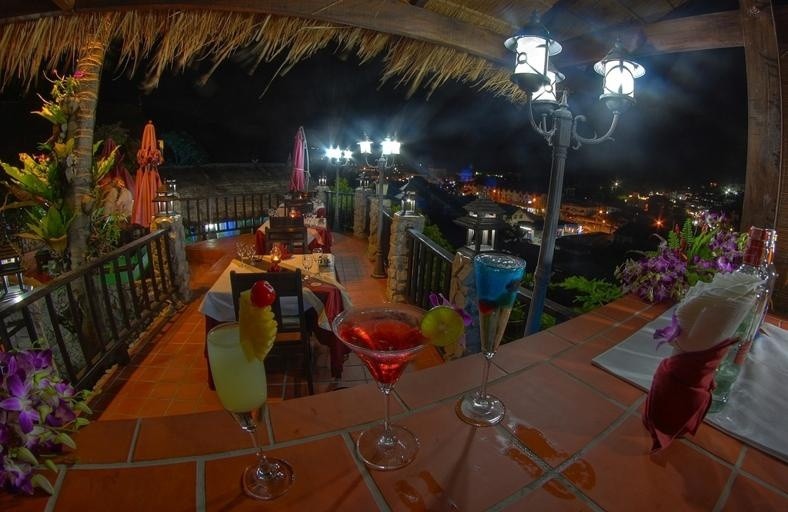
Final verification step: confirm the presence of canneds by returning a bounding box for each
[743,227,777,268]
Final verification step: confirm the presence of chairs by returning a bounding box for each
[200,188,350,396]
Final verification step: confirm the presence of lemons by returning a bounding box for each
[420,305,463,347]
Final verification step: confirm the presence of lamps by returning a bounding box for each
[356,167,371,188]
[452,189,502,253]
[152,170,179,216]
[397,177,420,215]
[316,169,330,187]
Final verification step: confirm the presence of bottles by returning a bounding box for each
[707,225,780,415]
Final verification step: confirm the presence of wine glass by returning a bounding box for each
[207,322,297,501]
[235,242,324,284]
[332,301,438,471]
[456,253,526,429]
[266,207,318,227]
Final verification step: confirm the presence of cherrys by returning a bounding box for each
[250,282,275,307]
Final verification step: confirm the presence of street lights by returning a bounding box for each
[322,143,354,230]
[355,130,406,279]
[496,7,651,347]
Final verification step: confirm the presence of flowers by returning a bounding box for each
[0,339,97,497]
[617,209,740,307]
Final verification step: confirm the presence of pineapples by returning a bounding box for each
[239,290,278,362]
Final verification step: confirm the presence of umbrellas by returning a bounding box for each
[94,137,135,229]
[130,120,166,229]
[300,126,312,192]
[288,127,305,192]
